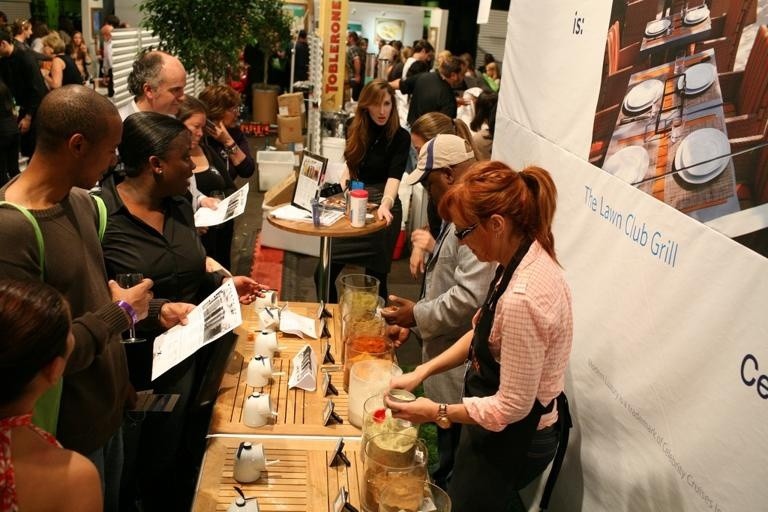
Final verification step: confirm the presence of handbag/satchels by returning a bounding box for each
[32,373,66,438]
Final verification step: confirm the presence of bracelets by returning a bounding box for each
[113,300,137,327]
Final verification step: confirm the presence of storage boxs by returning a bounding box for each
[276,90,307,117]
[263,169,296,207]
[276,138,307,150]
[259,199,321,259]
[276,114,304,143]
[255,150,297,192]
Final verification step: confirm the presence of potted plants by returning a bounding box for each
[251,6,293,122]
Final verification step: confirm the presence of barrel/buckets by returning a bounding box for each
[321,137,347,162]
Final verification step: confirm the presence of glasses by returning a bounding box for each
[454,218,486,243]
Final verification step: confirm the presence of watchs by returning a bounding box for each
[434,401,451,430]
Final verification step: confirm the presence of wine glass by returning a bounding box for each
[113,272,149,344]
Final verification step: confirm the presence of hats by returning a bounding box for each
[405,132,475,188]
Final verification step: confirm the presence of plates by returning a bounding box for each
[599,128,731,190]
[622,63,719,114]
[644,9,711,37]
[377,22,403,42]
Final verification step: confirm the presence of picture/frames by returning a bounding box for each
[374,17,406,47]
[429,26,438,50]
[289,149,331,213]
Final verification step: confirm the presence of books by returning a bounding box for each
[149,279,242,384]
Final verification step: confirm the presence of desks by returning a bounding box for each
[189,436,437,511]
[208,300,400,435]
[265,197,392,301]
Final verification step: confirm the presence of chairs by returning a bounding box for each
[516,390,571,511]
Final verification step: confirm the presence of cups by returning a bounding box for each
[338,274,450,512]
[674,49,685,74]
[310,197,326,228]
[351,187,370,230]
[671,118,686,142]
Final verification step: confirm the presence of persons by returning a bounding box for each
[88,109,271,485]
[0,85,198,512]
[382,159,575,512]
[0,274,108,511]
[378,132,501,488]
